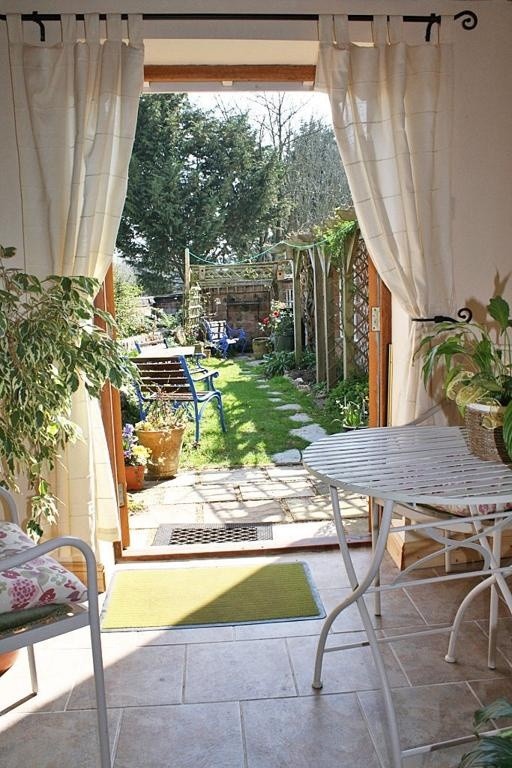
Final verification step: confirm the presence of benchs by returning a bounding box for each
[204,317,247,355]
[125,338,229,444]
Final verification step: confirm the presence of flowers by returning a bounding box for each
[263,298,296,336]
[119,424,153,467]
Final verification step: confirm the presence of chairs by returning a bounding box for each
[0,489,114,767]
[372,340,512,672]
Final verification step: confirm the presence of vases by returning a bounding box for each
[124,463,145,490]
[273,333,293,352]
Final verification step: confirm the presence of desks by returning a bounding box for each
[289,424,512,768]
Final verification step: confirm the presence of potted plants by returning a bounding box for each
[413,286,512,461]
[134,381,187,479]
[0,244,140,678]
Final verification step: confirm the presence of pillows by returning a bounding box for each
[0,518,89,629]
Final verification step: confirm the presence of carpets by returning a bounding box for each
[97,558,328,627]
